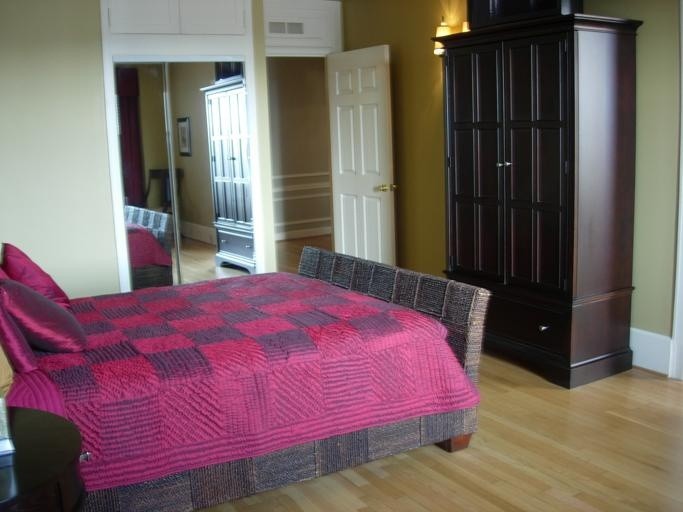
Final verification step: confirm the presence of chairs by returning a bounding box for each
[139,169,184,213]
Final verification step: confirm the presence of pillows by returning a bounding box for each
[1,242,88,374]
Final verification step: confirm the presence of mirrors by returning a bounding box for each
[116,62,266,292]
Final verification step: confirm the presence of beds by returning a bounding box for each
[125,205,173,289]
[0,247,495,512]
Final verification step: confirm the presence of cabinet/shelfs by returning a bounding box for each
[99,1,252,55]
[199,61,256,277]
[430,1,643,391]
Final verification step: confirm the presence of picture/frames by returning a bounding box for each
[176,117,192,157]
